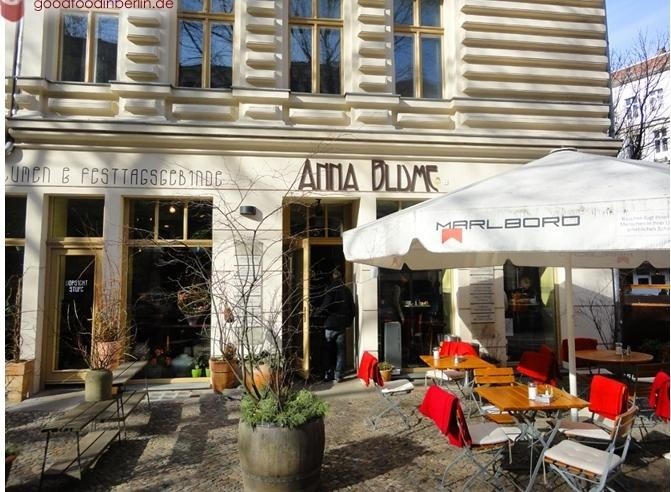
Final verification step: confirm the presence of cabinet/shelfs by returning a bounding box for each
[41,397,120,488]
[93,361,150,443]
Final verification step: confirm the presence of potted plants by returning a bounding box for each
[5,445,22,488]
[378,360,392,381]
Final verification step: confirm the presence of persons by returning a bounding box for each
[384,272,409,325]
[513,275,541,303]
[318,267,356,382]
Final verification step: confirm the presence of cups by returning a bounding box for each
[419,302,423,306]
[406,300,411,305]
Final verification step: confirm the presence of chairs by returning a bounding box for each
[425,339,670,492]
[365,349,414,430]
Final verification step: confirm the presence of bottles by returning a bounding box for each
[545,384,553,398]
[615,343,622,356]
[626,345,631,355]
[432,347,442,359]
[527,382,538,400]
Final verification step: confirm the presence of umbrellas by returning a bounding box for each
[342,146,670,424]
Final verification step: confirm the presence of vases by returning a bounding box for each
[239,416,325,492]
[5,297,286,403]
